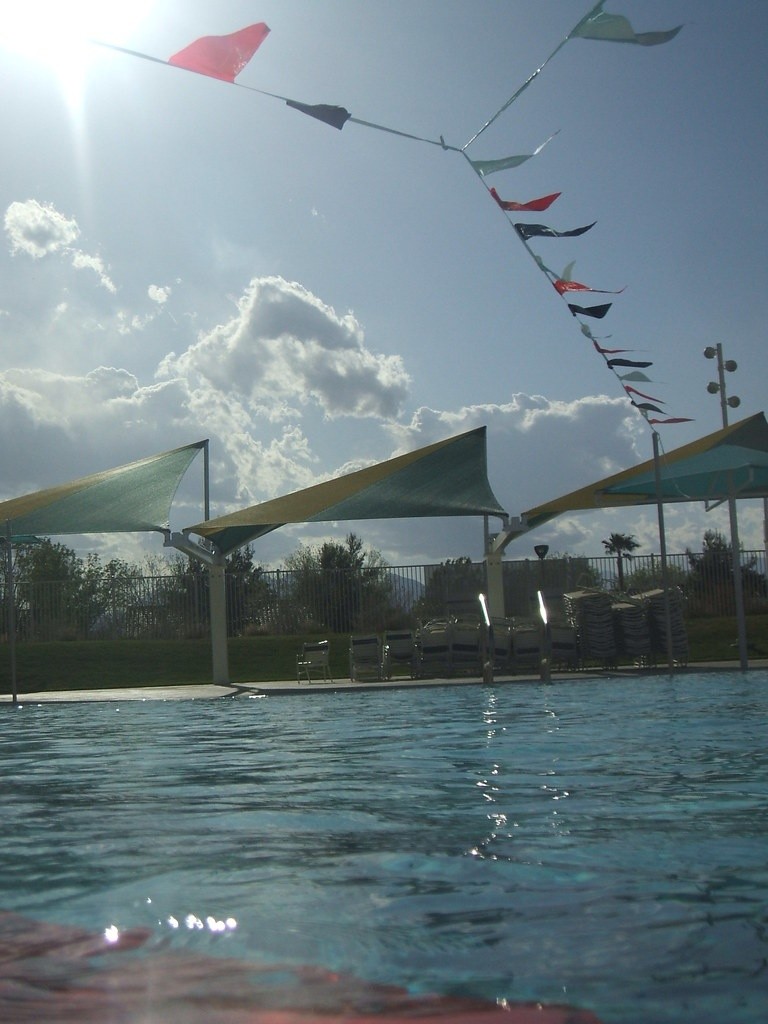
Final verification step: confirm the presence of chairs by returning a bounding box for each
[295,640,335,685]
[348,585,691,684]
